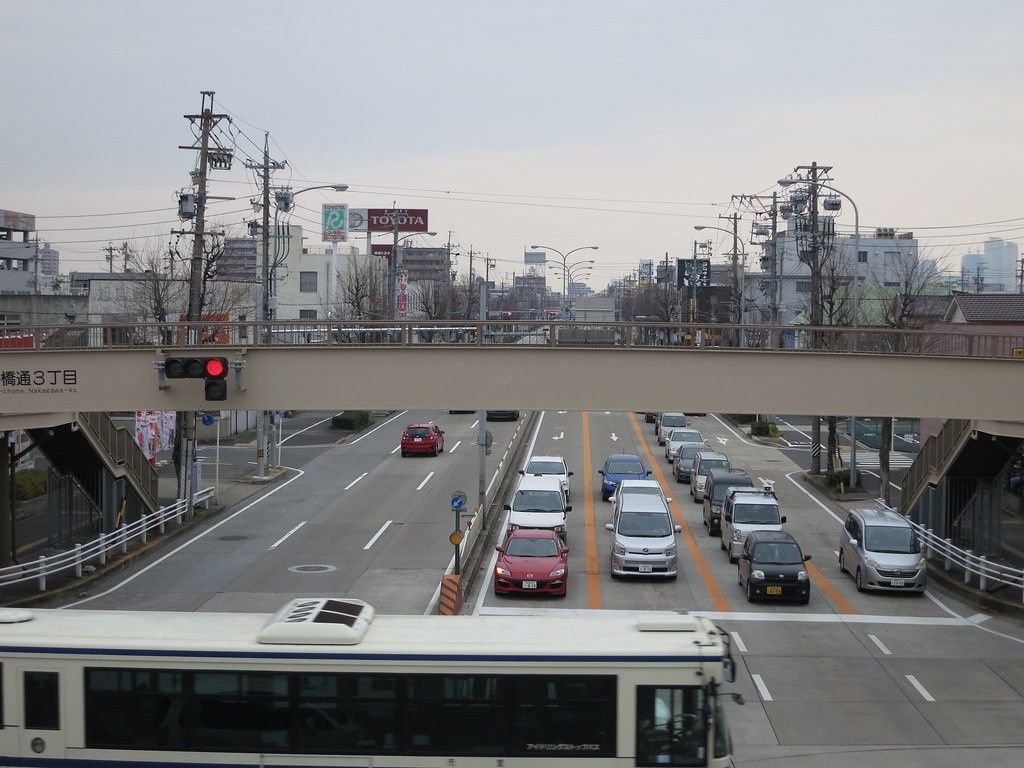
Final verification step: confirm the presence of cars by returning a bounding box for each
[671,442,715,483]
[487,410,519,421]
[516,453,575,503]
[598,452,653,502]
[490,528,571,599]
[608,480,674,511]
[638,411,709,465]
[400,422,446,458]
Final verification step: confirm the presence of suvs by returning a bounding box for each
[837,506,928,596]
[604,492,682,580]
[503,475,574,544]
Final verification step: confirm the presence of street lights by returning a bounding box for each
[530,245,599,328]
[543,258,595,319]
[270,183,351,472]
[386,230,440,343]
[777,179,859,488]
[549,265,594,311]
[694,225,745,348]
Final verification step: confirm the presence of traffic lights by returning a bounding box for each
[550,312,559,315]
[164,354,229,403]
[502,312,511,316]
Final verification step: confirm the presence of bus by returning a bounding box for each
[0,599,749,767]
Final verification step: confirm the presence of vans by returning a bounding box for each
[738,530,814,605]
[720,486,788,564]
[690,451,733,503]
[702,468,756,537]
